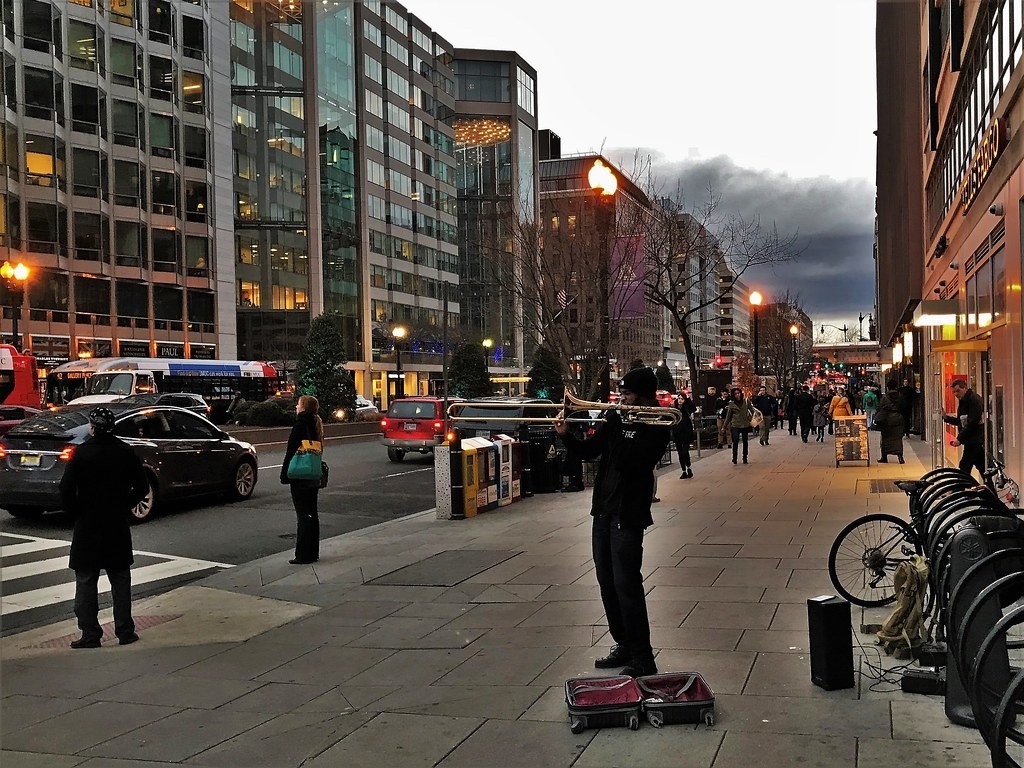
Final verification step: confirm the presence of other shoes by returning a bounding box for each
[728,445,732,448]
[743,458,747,463]
[765,442,769,445]
[289,557,317,564]
[70,639,102,648]
[679,472,688,479]
[119,632,139,645]
[732,459,737,464]
[877,456,887,463]
[687,468,693,478]
[717,444,723,449]
[652,497,660,503]
[788,428,824,444]
[899,456,905,464]
[760,439,764,446]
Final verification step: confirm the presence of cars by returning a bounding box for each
[355,394,379,415]
[579,392,621,436]
[0,402,258,527]
[656,389,693,408]
[0,404,43,439]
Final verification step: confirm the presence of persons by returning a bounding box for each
[715,388,732,449]
[878,378,904,464]
[702,386,721,449]
[786,377,881,443]
[721,388,761,465]
[774,391,785,430]
[57,407,150,649]
[550,367,672,680]
[942,378,998,496]
[745,391,759,436]
[673,392,696,480]
[279,396,323,564]
[897,378,919,440]
[226,391,246,413]
[753,387,776,446]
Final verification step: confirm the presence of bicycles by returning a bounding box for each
[826,451,1024,609]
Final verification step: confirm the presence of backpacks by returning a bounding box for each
[876,555,929,653]
[867,396,874,406]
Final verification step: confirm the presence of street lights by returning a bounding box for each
[482,339,493,372]
[749,290,763,375]
[821,323,848,342]
[790,324,799,391]
[858,311,874,342]
[0,260,30,354]
[588,159,617,402]
[392,326,407,400]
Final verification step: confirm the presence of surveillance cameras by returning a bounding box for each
[949,262,959,270]
[989,203,1003,216]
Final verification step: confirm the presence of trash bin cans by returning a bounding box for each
[517,430,559,494]
[700,415,718,445]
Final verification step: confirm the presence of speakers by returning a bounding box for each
[807,596,855,691]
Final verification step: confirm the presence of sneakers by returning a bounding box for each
[618,663,657,678]
[592,644,630,668]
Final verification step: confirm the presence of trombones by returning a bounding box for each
[445,386,683,427]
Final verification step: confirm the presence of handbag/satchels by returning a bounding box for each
[745,398,763,427]
[286,450,323,480]
[317,460,329,489]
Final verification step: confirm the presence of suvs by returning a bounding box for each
[446,397,560,474]
[378,395,468,463]
[119,392,211,422]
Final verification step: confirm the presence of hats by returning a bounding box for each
[619,367,658,398]
[89,407,116,432]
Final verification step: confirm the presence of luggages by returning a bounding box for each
[564,672,715,734]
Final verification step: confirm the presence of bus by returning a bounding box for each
[0,343,42,411]
[41,357,277,409]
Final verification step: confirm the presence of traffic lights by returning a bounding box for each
[839,363,844,372]
[824,363,830,369]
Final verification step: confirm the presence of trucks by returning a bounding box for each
[60,370,288,425]
